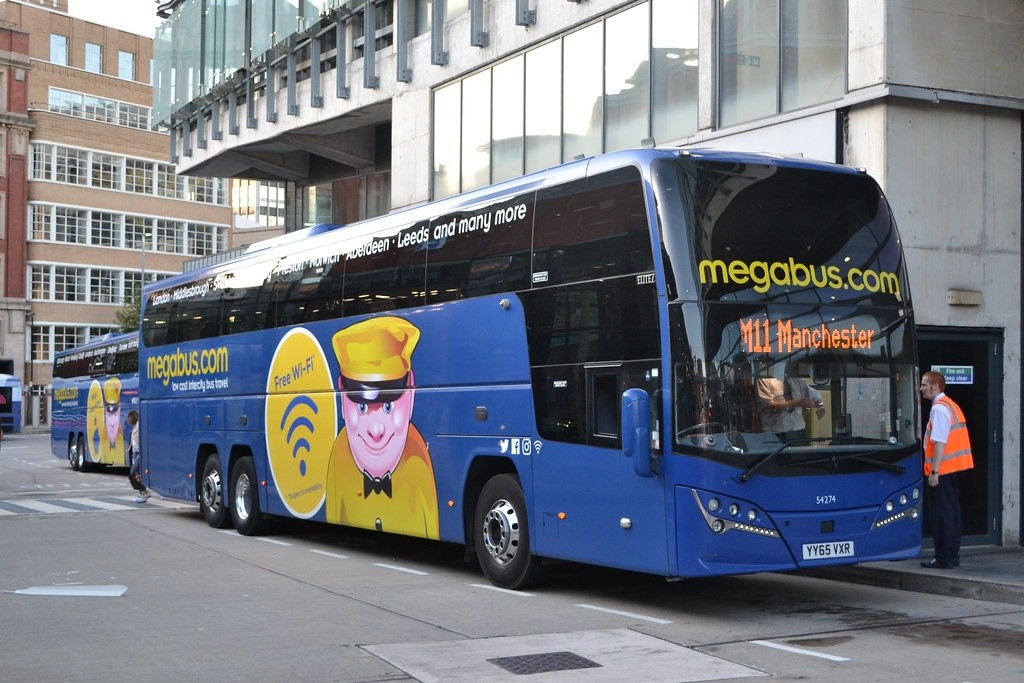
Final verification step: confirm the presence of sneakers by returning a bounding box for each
[133,491,152,503]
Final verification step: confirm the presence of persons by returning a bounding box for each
[755,377,826,447]
[125,410,152,503]
[919,371,975,570]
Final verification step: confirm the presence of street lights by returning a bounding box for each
[29,313,35,397]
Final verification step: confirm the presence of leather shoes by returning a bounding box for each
[920,555,960,568]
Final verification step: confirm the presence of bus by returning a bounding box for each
[49,330,144,469]
[141,146,925,586]
[0,373,23,433]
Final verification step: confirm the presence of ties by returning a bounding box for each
[783,376,796,414]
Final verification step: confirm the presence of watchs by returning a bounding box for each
[931,471,939,474]
[816,402,824,406]
